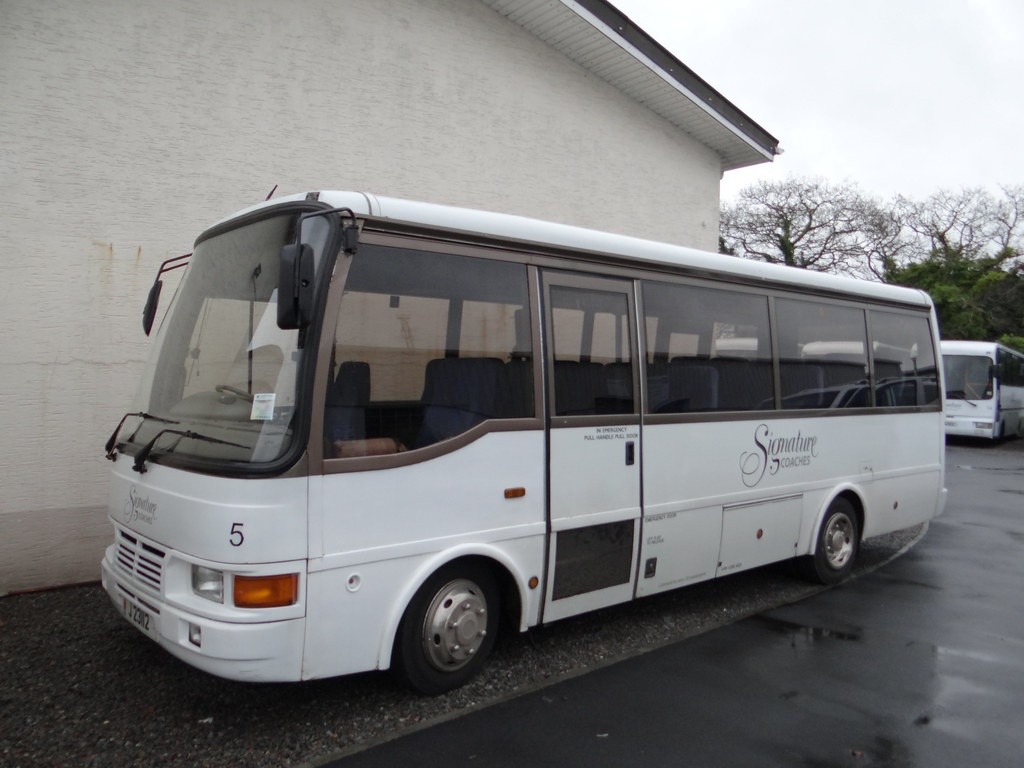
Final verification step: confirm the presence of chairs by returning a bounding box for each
[322,344,944,455]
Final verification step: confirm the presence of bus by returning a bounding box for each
[98,184,946,698]
[937,340,1023,445]
